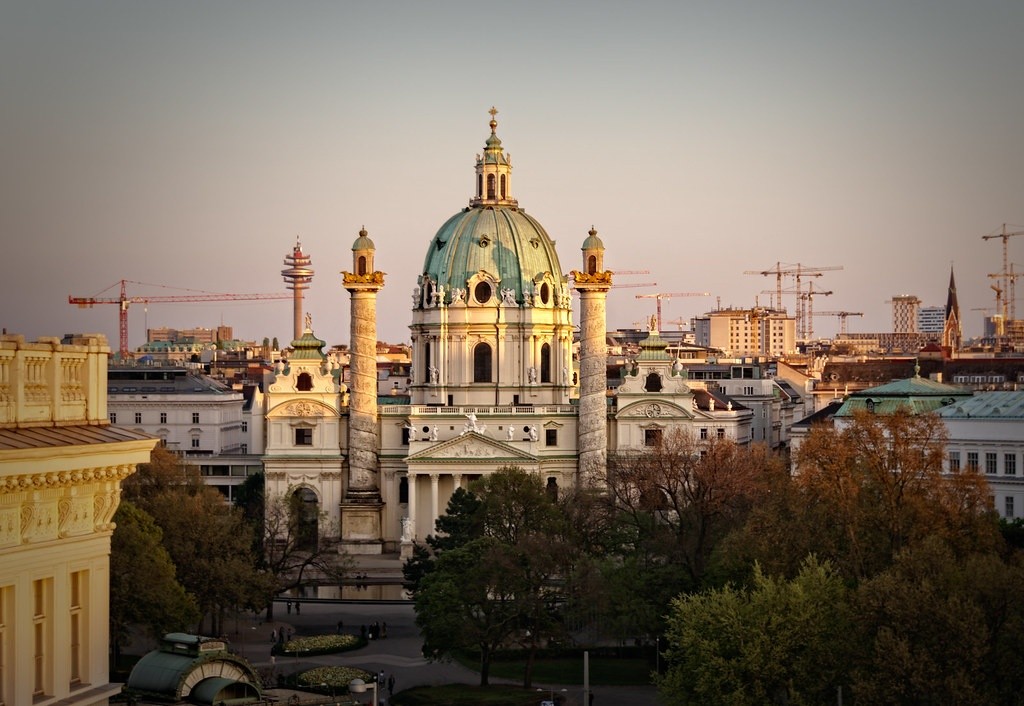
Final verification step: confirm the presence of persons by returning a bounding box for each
[380,669,386,691]
[388,674,395,695]
[405,412,538,443]
[450,288,463,305]
[502,288,516,306]
[428,366,439,382]
[277,671,297,691]
[269,625,292,664]
[287,598,300,615]
[336,620,387,642]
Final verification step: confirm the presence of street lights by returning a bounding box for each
[349,679,377,706]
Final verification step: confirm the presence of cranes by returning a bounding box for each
[970,222,1024,319]
[68,280,305,362]
[788,311,864,334]
[633,316,688,332]
[743,261,844,340]
[635,292,712,331]
